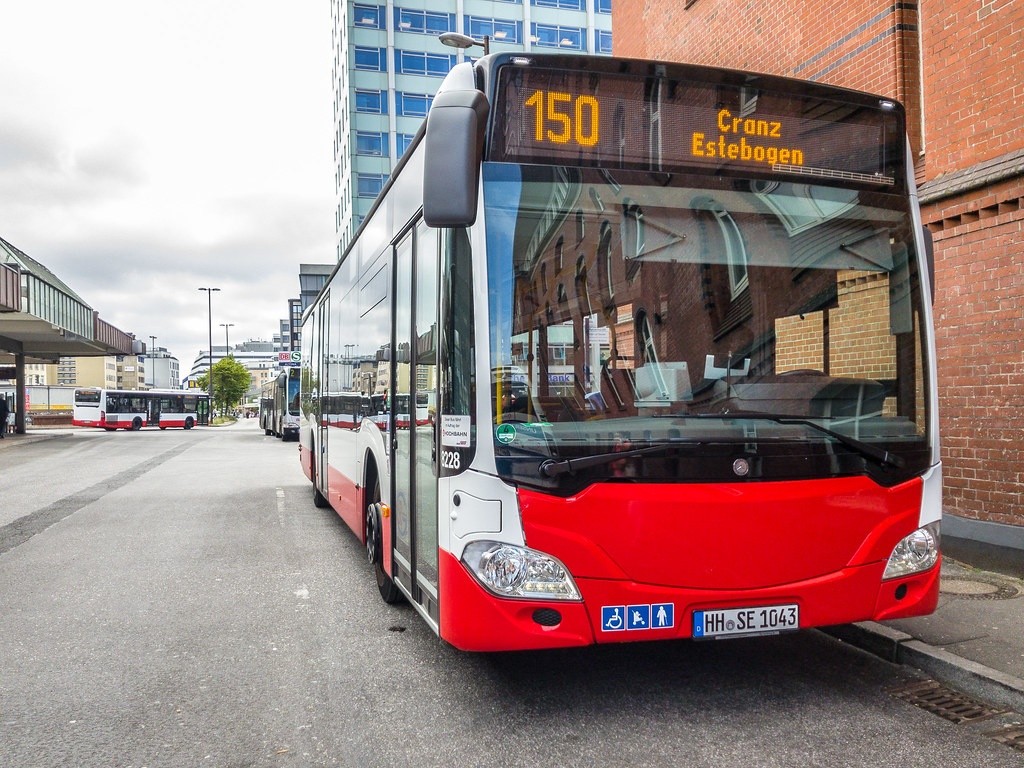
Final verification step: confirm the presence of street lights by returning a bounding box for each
[199,288,220,423]
[150,336,157,388]
[438,32,489,57]
[220,324,235,356]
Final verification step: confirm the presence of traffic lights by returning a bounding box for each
[344,344,354,392]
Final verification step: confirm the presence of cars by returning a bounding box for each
[222,408,226,413]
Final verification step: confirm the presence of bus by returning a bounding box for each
[319,393,428,429]
[299,52,944,652]
[258,366,300,442]
[72,386,209,431]
